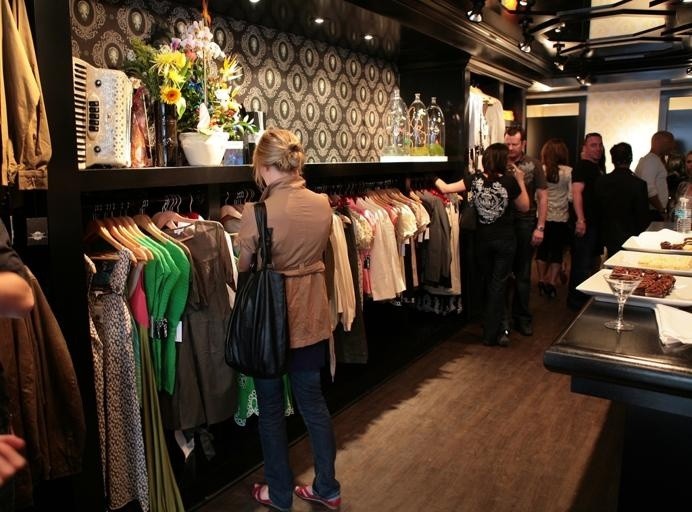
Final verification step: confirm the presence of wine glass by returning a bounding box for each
[603,269,643,332]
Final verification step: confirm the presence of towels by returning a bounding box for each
[653,305,692,345]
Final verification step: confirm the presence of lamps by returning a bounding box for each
[467,0,594,86]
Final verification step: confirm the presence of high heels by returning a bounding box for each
[546,282,556,297]
[537,280,546,295]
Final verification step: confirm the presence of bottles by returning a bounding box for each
[380,85,407,155]
[424,95,447,156]
[406,93,429,156]
[674,197,692,232]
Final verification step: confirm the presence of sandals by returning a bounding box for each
[293,482,342,509]
[251,482,292,511]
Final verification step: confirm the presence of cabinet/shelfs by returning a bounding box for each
[469,56,533,323]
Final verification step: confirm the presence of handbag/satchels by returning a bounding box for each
[222,201,290,380]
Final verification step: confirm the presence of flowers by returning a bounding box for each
[129,21,256,141]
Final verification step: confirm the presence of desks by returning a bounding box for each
[543,221,692,512]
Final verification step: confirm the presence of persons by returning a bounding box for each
[596,142,651,270]
[633,128,677,223]
[565,132,611,312]
[232,126,344,510]
[544,238,570,286]
[664,149,688,214]
[669,151,692,223]
[499,124,551,338]
[533,138,572,298]
[428,143,533,347]
[1,214,41,483]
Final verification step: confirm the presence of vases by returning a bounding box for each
[178,132,230,166]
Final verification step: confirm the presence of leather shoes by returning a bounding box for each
[498,332,510,345]
[516,319,534,335]
[483,334,498,346]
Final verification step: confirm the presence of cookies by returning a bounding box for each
[607,233,692,297]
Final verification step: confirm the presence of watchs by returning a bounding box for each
[535,225,545,231]
[576,219,587,224]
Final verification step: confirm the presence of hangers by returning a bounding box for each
[322,176,463,224]
[82,188,255,263]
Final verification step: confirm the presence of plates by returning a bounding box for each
[620,233,692,253]
[604,250,692,275]
[574,267,692,312]
